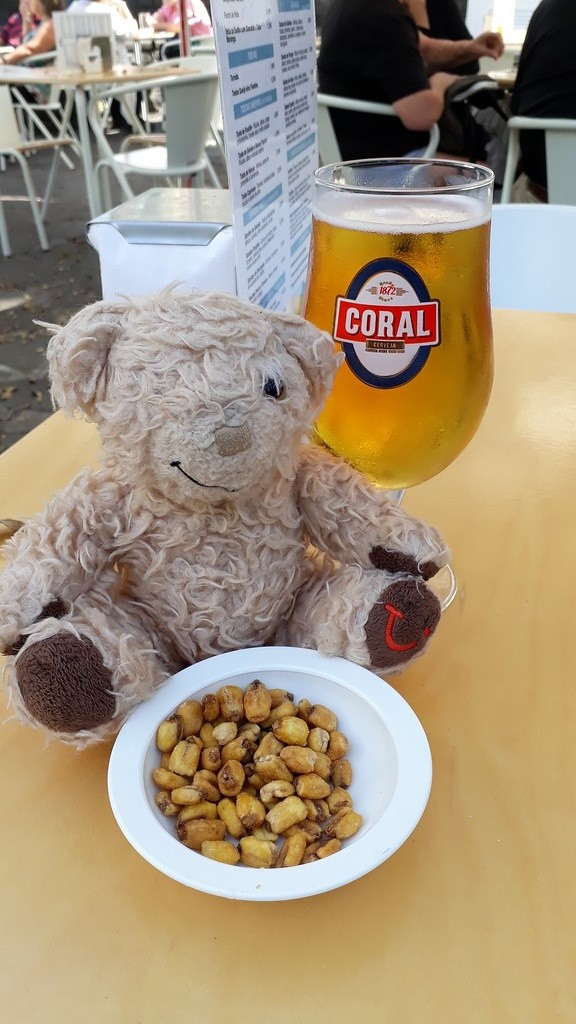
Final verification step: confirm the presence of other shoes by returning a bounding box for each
[527,180,548,202]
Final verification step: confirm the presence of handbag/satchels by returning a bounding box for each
[444,75,512,140]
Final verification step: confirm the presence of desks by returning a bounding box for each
[0,64,197,240]
[0,308,576,1024]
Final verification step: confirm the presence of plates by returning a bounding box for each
[106,644,432,902]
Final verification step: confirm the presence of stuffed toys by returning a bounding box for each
[0,280,451,753]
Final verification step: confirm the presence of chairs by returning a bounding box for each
[0,8,230,258]
[308,96,576,204]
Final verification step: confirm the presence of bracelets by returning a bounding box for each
[1,55,6,64]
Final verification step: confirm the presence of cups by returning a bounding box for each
[138,12,150,29]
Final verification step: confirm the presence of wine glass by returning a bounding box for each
[299,156,496,611]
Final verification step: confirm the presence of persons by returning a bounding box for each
[63,0,155,132]
[150,0,212,60]
[0,0,68,140]
[317,0,505,190]
[509,0,576,204]
